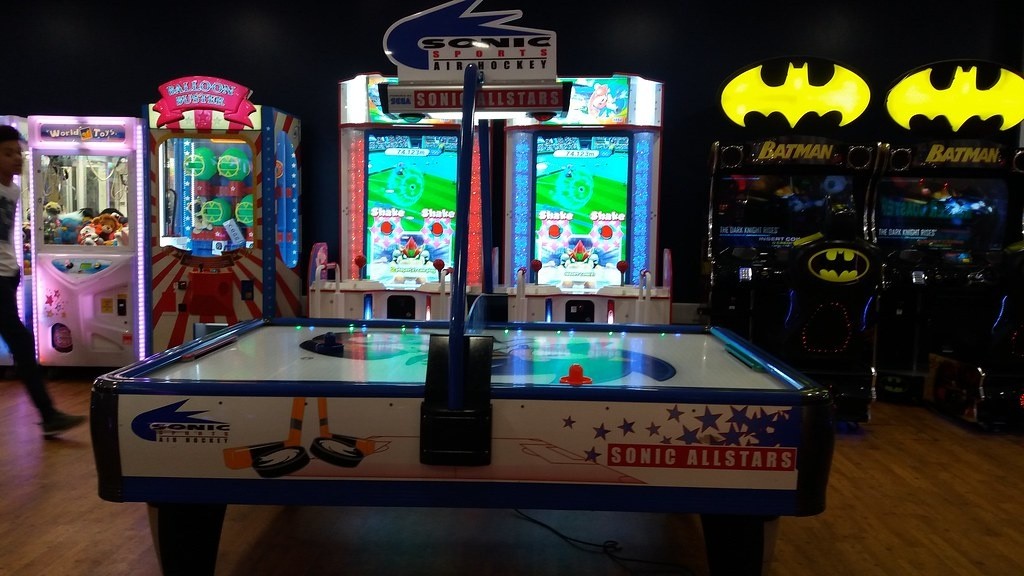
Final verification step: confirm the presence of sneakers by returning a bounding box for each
[41,407,88,436]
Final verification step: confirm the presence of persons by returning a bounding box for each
[0,124,86,435]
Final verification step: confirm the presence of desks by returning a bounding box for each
[90,316,837,576]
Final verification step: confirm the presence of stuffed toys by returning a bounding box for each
[22,208,31,243]
[43,201,128,247]
